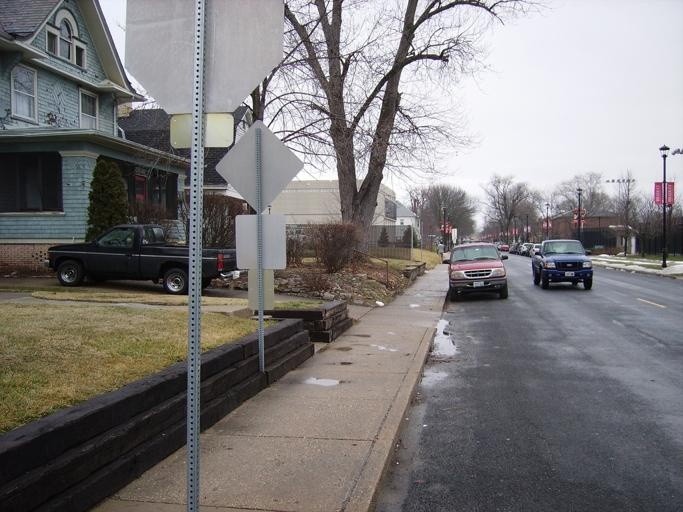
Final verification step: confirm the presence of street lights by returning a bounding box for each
[659,144,670,268]
[576,187,583,239]
[545,202,550,239]
[526,212,529,239]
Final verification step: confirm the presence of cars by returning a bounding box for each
[442,239,593,302]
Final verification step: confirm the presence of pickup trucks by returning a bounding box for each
[46,224,236,295]
